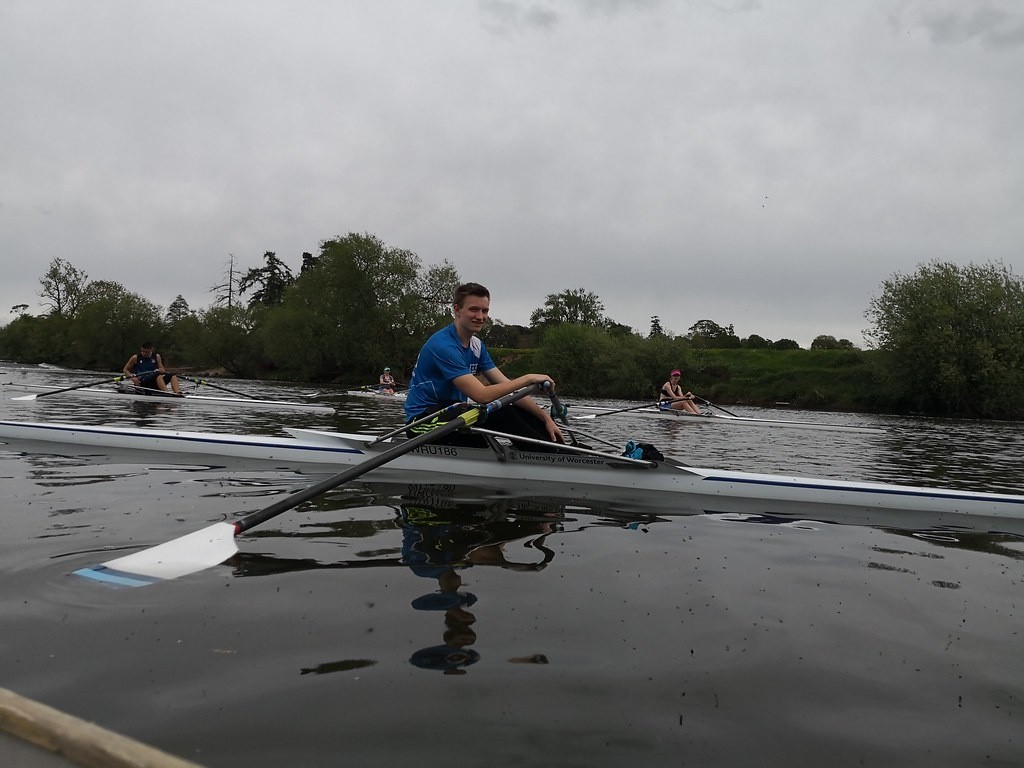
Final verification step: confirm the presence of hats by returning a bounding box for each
[384,367,390,371]
[671,370,681,377]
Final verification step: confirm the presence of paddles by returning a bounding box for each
[546,384,593,451]
[574,396,692,419]
[72,381,546,592]
[12,370,155,400]
[164,372,263,400]
[340,382,389,393]
[695,394,739,417]
[393,381,409,389]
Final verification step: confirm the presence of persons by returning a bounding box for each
[658,369,701,414]
[379,367,395,393]
[123,341,179,393]
[404,283,566,453]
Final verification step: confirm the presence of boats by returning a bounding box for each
[566,404,889,437]
[347,389,407,403]
[2,379,338,417]
[0,417,1024,536]
